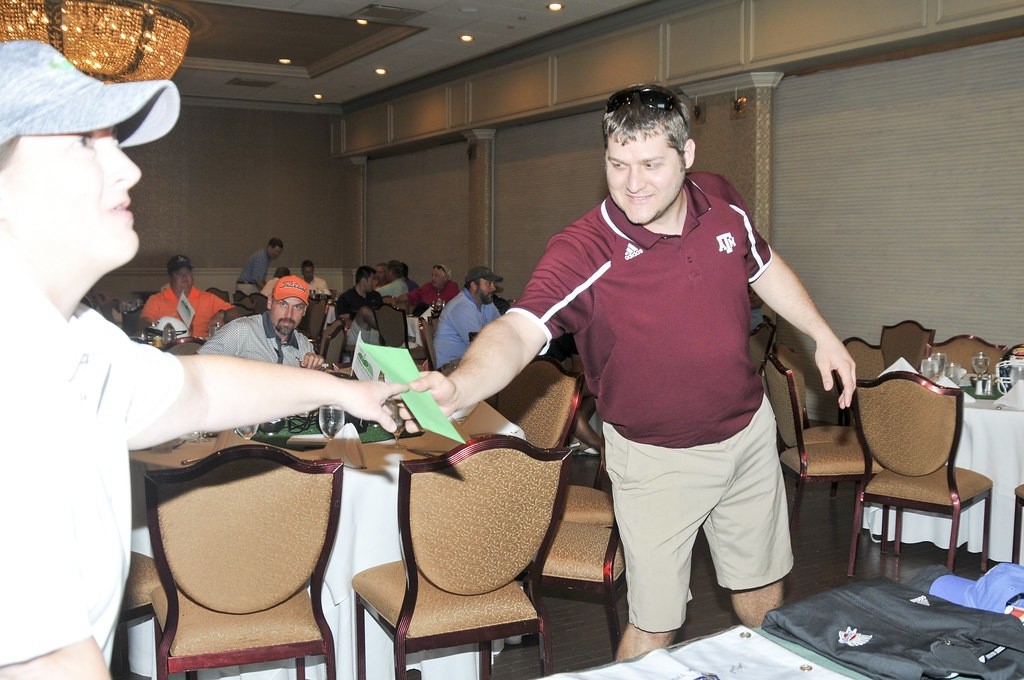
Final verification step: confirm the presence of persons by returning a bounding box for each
[234,237,284,296]
[300,259,332,295]
[335,264,385,345]
[259,267,291,310]
[375,260,410,298]
[747,283,766,332]
[193,274,325,371]
[373,262,388,290]
[381,263,461,318]
[409,85,859,662]
[0,40,408,680]
[545,333,603,453]
[433,267,504,377]
[132,254,237,340]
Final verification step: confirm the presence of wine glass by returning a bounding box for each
[973,351,990,382]
[386,399,406,450]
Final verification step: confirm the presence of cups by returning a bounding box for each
[318,406,345,440]
[162,331,176,344]
[235,424,259,441]
[208,327,220,338]
[1011,367,1024,386]
[920,352,961,387]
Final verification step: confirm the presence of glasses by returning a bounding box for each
[605,88,689,130]
[433,264,447,275]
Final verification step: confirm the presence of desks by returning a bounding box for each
[124,400,527,680]
[864,374,1024,564]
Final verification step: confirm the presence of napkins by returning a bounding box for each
[212,430,244,454]
[318,422,367,468]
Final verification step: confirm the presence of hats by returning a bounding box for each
[271,274,312,306]
[166,254,194,276]
[465,265,504,283]
[0,39,182,149]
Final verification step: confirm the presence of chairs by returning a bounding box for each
[83,288,626,680]
[750,314,1024,573]
